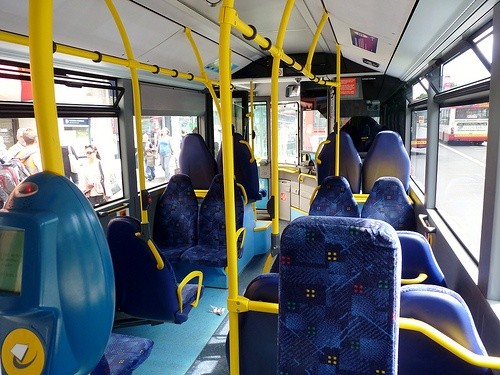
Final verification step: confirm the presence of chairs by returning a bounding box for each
[87,133,272,375]
[225,130,494,375]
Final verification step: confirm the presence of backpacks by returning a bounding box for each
[0,150,39,197]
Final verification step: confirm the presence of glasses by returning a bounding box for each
[86,150,95,155]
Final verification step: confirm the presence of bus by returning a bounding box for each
[304,110,352,159]
[410,110,427,152]
[439,102,489,145]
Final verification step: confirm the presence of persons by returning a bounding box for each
[62,146,79,183]
[143,127,192,181]
[79,146,112,206]
[0,129,42,209]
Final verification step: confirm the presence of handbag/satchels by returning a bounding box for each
[175,161,180,174]
[98,160,121,197]
